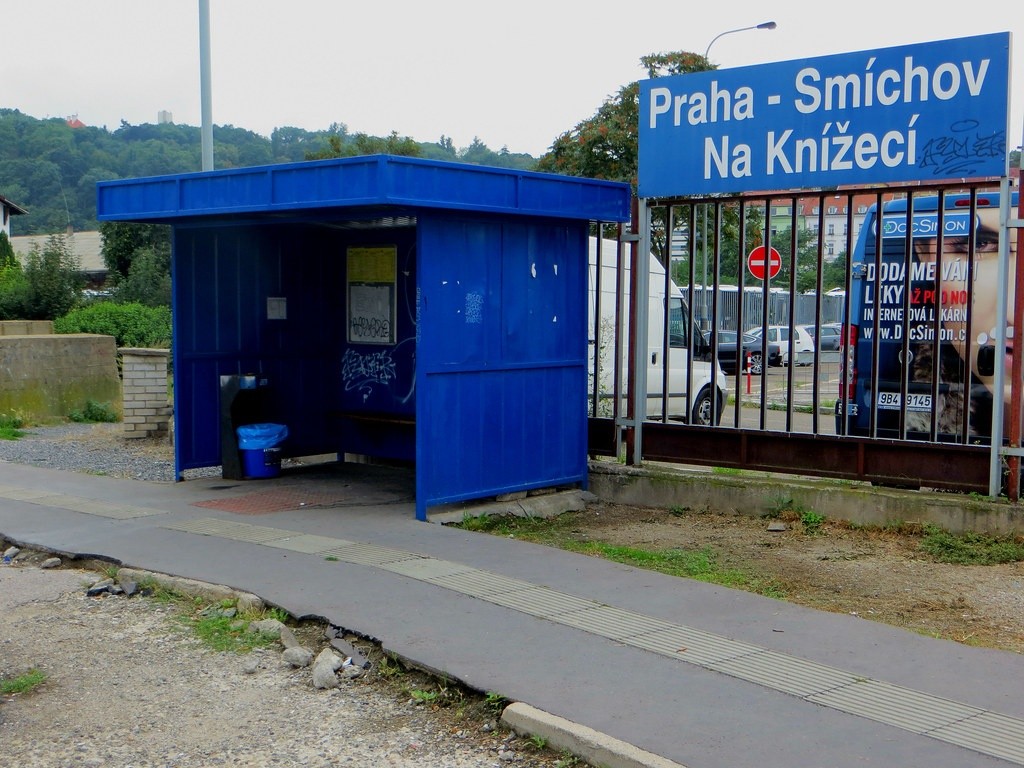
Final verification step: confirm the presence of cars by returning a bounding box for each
[695,328,780,376]
[745,326,815,368]
[800,324,843,351]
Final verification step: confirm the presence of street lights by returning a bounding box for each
[700,22,776,332]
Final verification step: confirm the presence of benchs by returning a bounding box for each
[269,399,416,482]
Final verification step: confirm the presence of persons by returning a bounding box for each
[912,207,1022,436]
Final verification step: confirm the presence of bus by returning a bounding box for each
[838,192,1020,447]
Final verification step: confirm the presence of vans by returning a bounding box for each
[588,237,728,427]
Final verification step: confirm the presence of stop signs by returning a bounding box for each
[747,245,782,282]
